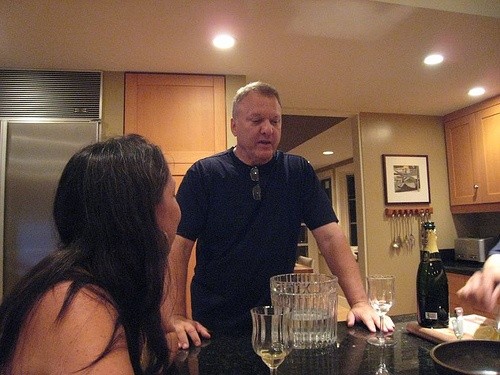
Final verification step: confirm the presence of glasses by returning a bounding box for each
[249,165,263,201]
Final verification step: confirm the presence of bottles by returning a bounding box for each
[416,221,449,328]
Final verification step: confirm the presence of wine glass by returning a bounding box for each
[371,345,391,375]
[366,275,398,346]
[250,306,294,375]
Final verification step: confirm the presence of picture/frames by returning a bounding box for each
[381,153,432,205]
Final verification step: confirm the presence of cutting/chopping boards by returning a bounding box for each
[406,313,500,341]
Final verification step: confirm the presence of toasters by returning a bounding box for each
[454,235,494,266]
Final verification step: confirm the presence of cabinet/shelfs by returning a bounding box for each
[447,272,500,321]
[442,94,500,215]
[295,168,333,276]
[123,70,227,321]
[332,161,358,299]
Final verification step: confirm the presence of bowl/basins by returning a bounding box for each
[430,340,500,375]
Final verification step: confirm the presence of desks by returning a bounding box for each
[173,311,458,375]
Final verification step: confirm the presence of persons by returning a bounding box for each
[1,134,182,375]
[456,240,500,311]
[160,82,396,350]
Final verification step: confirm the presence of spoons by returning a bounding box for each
[392,212,415,248]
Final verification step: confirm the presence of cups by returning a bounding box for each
[276,348,339,375]
[270,273,338,350]
